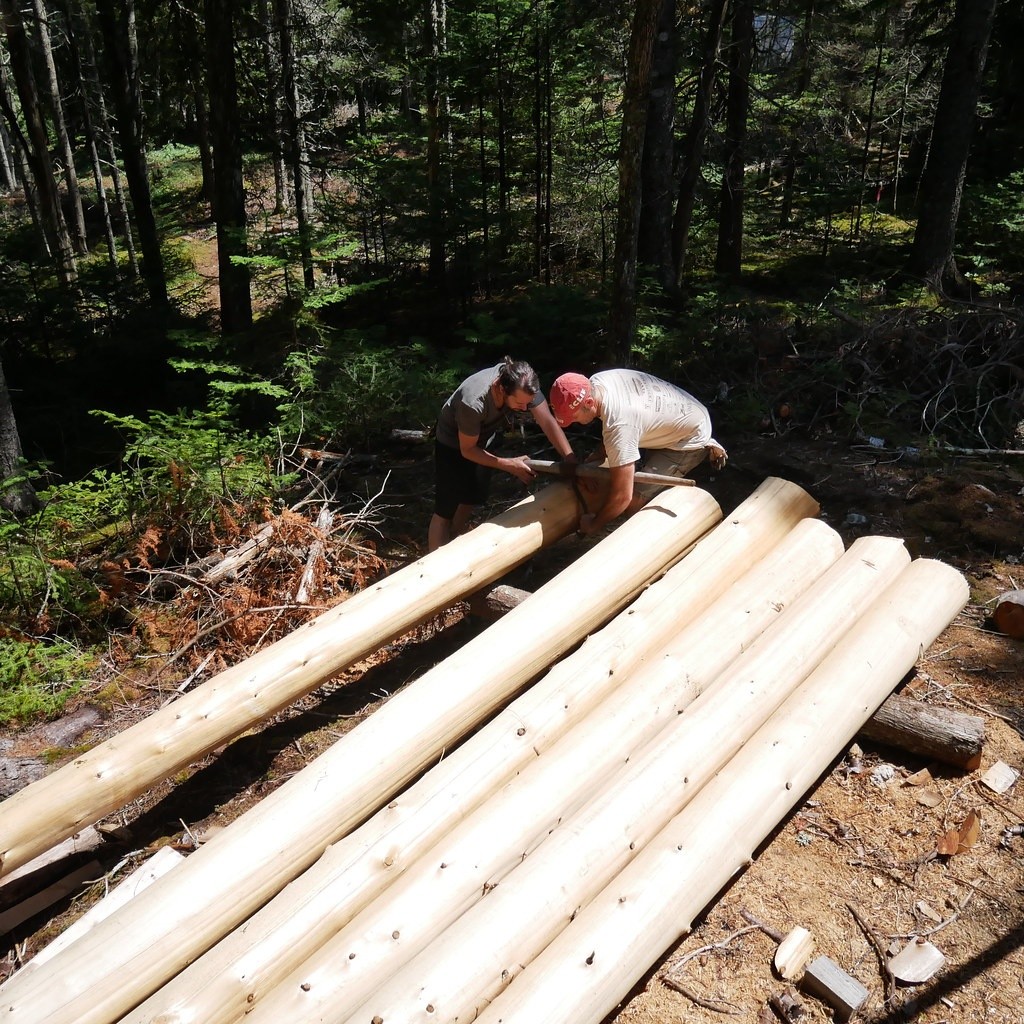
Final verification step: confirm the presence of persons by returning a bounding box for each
[428,356,598,554]
[550,369,712,537]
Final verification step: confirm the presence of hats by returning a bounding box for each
[550,373,591,427]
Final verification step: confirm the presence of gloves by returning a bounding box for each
[704,438,729,471]
